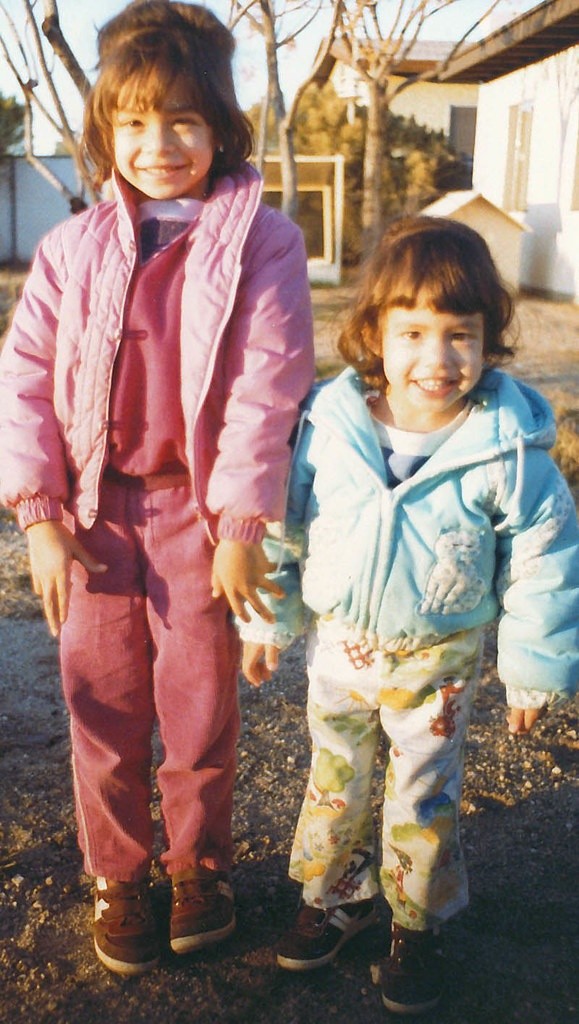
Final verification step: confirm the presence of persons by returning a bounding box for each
[0,0,312,979]
[229,214,579,1017]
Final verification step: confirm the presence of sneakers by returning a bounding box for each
[93,876,160,973]
[381,922,447,1015]
[169,869,236,954]
[276,899,375,971]
